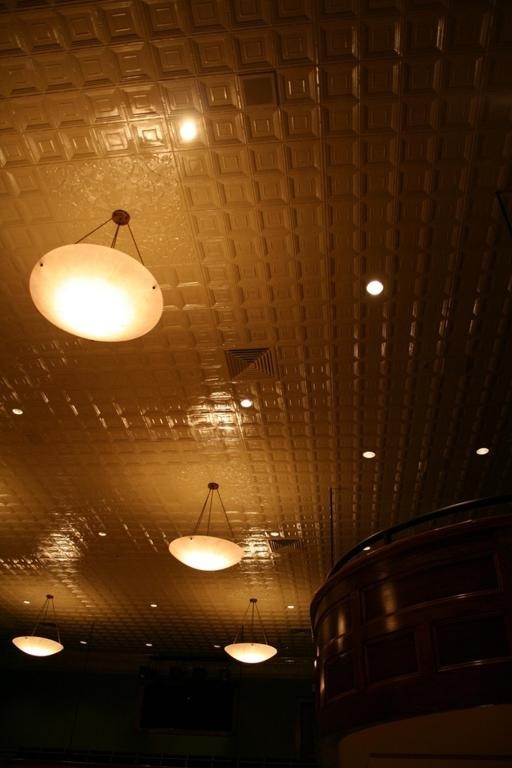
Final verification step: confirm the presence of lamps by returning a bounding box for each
[170,481,244,573]
[13,595,64,657]
[225,598,278,665]
[30,210,164,342]
